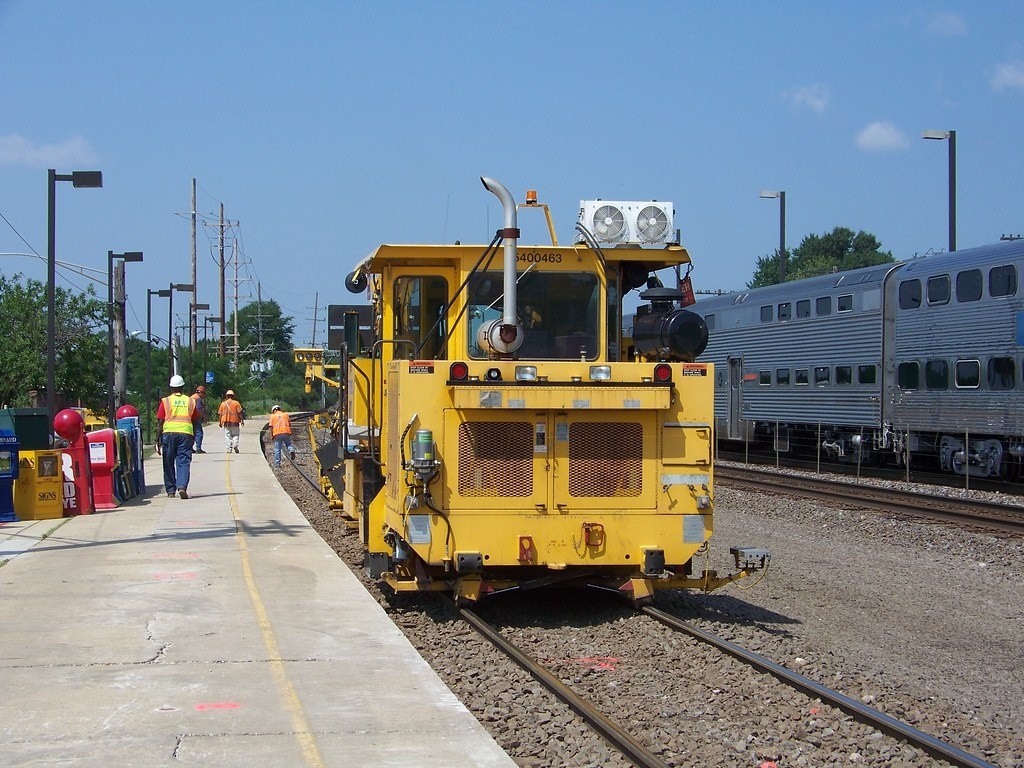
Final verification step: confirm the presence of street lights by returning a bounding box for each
[188,302,209,397]
[108,250,144,427]
[203,316,221,413]
[760,190,786,282]
[47,169,101,451]
[146,282,194,445]
[921,129,956,253]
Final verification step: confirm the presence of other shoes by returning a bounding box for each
[179,488,188,500]
[274,464,281,467]
[227,447,239,453]
[168,493,175,498]
[192,449,205,453]
[290,451,295,460]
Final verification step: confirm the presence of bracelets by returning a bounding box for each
[155,439,159,442]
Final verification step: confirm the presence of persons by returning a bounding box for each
[269,404,295,467]
[155,375,195,499]
[218,390,244,453]
[190,386,206,453]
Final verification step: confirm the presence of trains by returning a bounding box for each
[293,176,771,613]
[621,240,1024,482]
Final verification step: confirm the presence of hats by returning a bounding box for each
[227,390,233,394]
[272,405,279,410]
[197,386,205,392]
[170,375,185,387]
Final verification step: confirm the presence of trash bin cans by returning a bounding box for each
[0,405,146,523]
